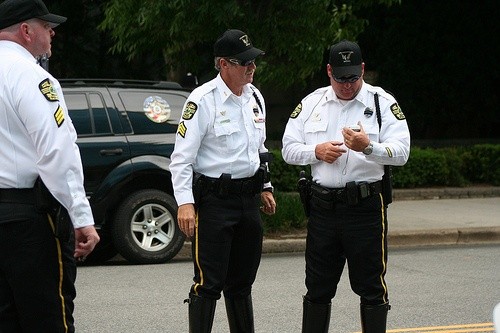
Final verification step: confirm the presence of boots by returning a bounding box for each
[183,293,216,333]
[360,299,391,332]
[302,294,332,333]
[225,294,254,332]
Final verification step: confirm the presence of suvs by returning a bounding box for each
[50,78,193,264]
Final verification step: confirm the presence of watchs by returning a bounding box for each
[363,139,374,154]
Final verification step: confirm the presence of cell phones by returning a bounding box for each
[352,127,361,132]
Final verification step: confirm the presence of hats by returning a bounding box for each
[0,0,67,29]
[214,29,265,61]
[329,40,362,77]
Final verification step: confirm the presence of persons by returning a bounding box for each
[0,0,100,333]
[280,40,411,332]
[168,29,276,333]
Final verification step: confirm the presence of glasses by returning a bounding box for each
[331,70,362,83]
[224,57,256,66]
